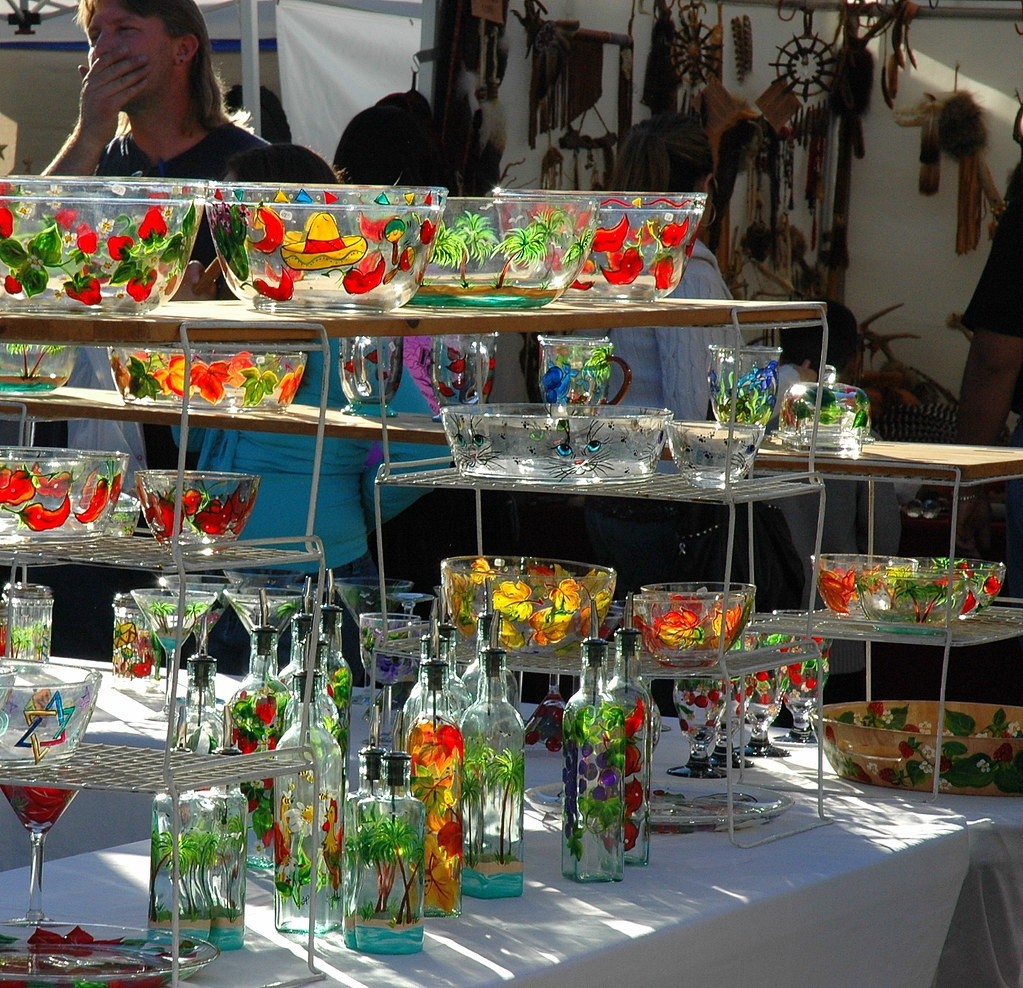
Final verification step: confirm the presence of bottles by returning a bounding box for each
[111,593,154,683]
[0,582,54,664]
[148,604,652,955]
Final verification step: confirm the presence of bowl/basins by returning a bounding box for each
[0,657,104,771]
[0,170,872,485]
[440,555,618,658]
[133,469,261,559]
[624,582,757,668]
[808,700,1023,797]
[811,553,1006,636]
[0,444,131,547]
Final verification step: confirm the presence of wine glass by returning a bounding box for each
[667,635,834,779]
[0,784,83,930]
[130,570,440,749]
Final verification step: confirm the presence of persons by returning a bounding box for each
[171,143,451,680]
[760,300,901,676]
[32,0,270,674]
[955,175,1023,608]
[575,114,818,601]
[332,104,568,507]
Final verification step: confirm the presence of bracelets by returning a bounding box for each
[959,495,975,500]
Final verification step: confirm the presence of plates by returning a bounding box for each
[0,921,223,988]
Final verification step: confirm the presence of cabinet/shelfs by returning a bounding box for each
[0,293,1023,988]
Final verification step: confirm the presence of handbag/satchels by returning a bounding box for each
[674,501,804,610]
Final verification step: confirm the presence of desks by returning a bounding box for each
[0,653,1023,988]
